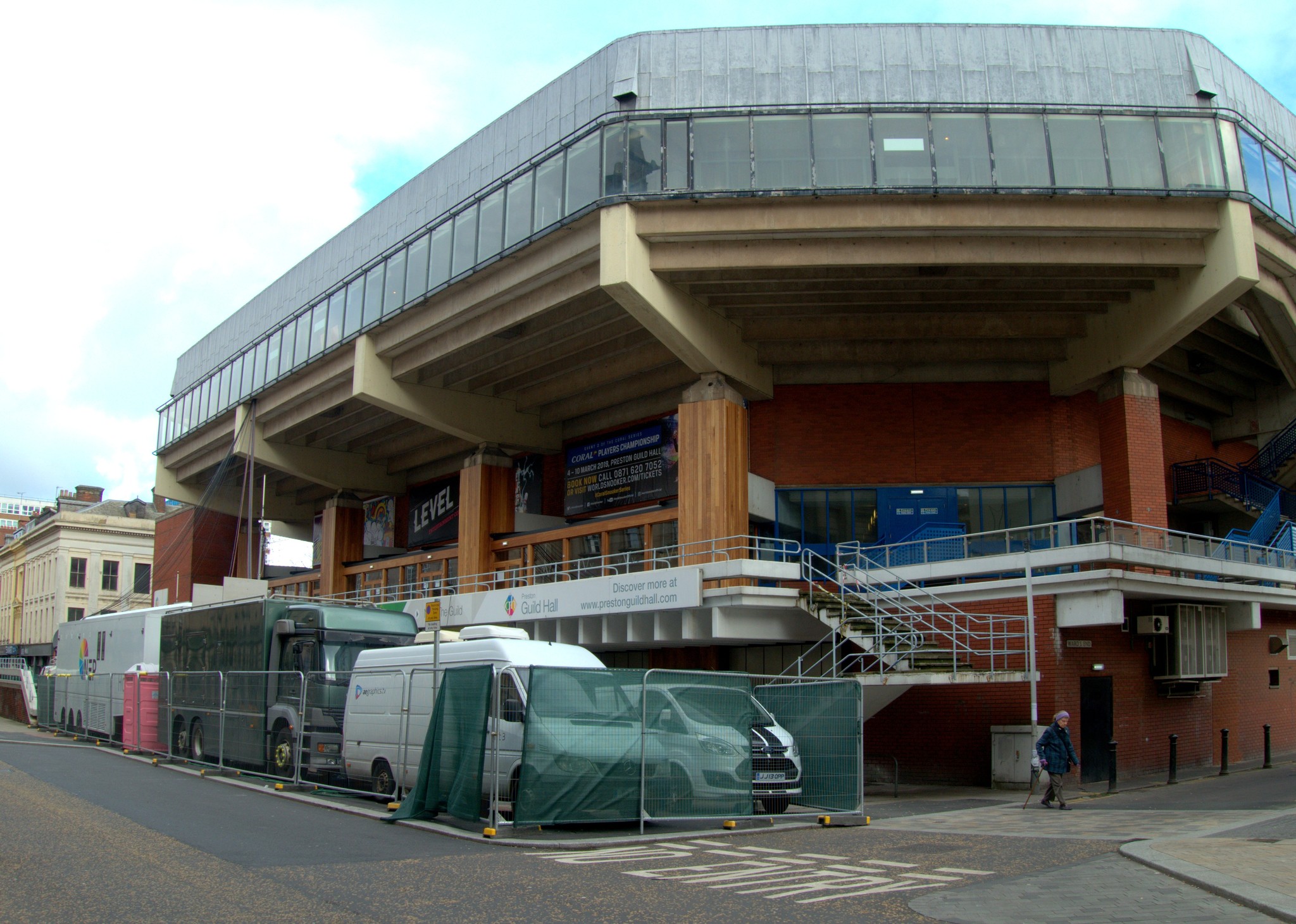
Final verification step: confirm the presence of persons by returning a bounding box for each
[1035,710,1080,810]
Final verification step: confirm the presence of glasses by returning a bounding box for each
[377,520,381,524]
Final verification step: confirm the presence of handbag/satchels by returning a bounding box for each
[1067,755,1071,772]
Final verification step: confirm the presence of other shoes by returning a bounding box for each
[1059,803,1072,810]
[1041,798,1055,808]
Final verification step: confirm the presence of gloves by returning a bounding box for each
[1040,759,1046,766]
[1075,763,1080,768]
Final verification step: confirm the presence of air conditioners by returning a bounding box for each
[1136,614,1169,634]
[1153,601,1229,681]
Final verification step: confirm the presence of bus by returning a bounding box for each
[49,600,192,743]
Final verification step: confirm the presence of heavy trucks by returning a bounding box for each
[158,593,419,785]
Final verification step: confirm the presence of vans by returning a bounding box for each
[340,625,668,823]
[592,682,802,816]
